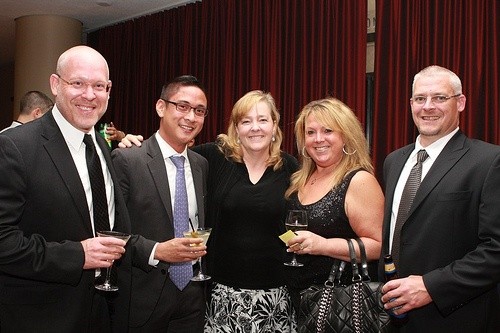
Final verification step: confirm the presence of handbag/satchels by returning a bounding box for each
[297,237,392,333]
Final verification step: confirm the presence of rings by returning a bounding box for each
[300,244,304,250]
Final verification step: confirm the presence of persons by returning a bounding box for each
[105,121,125,142]
[0,91,54,133]
[110,76,211,333]
[286,97,385,316]
[0,46,126,333]
[118,91,298,333]
[380,66,500,333]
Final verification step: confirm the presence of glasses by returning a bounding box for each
[160,97,208,118]
[56,73,110,94]
[412,94,461,108]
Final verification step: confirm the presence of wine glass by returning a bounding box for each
[182,227,212,281]
[94,230,131,292]
[284,210,308,266]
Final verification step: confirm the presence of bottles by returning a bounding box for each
[384,255,409,321]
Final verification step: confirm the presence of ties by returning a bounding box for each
[82,133,117,288]
[392,150,429,278]
[169,155,193,291]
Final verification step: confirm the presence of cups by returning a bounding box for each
[100,124,111,151]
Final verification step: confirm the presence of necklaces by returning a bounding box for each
[311,170,331,185]
